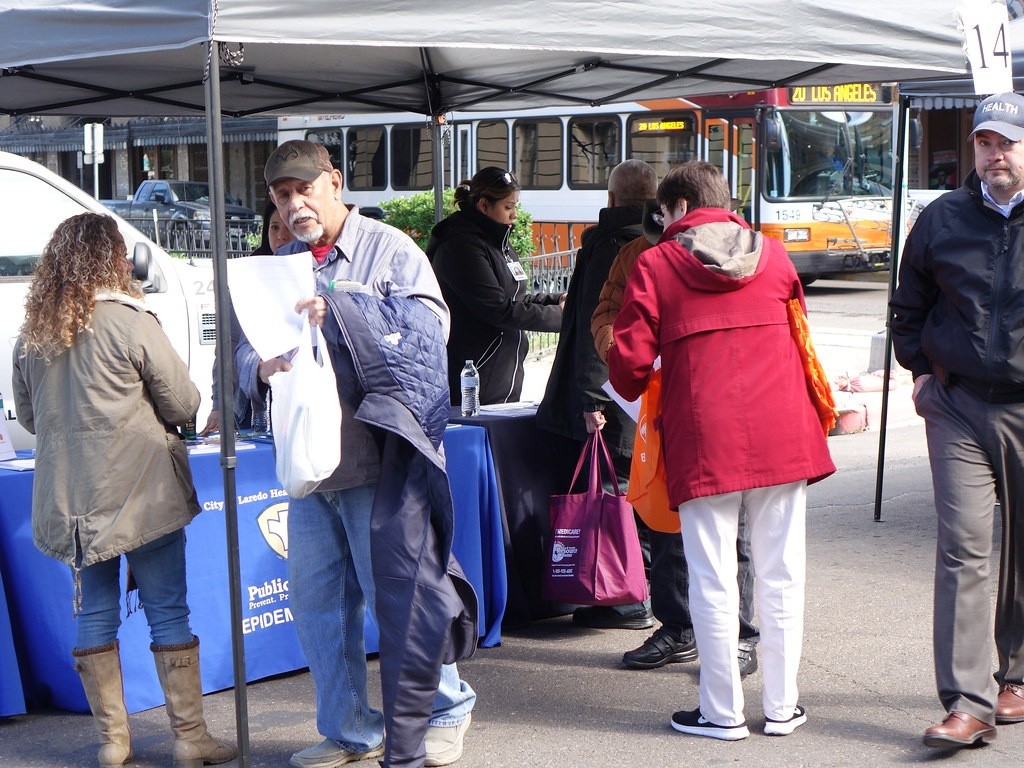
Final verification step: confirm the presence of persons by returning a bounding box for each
[930,167,955,190]
[816,138,843,170]
[14,211,238,768]
[608,158,838,740]
[197,203,297,439]
[235,141,477,768]
[425,167,568,406]
[525,159,653,629]
[885,88,1024,745]
[514,267,523,276]
[591,198,761,682]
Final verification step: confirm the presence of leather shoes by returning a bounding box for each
[623,626,698,668]
[923,712,997,747]
[738,641,758,679]
[573,606,656,629]
[996,684,1024,722]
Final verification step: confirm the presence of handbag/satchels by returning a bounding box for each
[542,427,650,606]
[625,366,681,534]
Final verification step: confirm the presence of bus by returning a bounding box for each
[275,85,925,290]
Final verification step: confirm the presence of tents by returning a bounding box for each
[873,15,1024,521]
[0,0,969,768]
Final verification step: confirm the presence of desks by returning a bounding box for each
[447,400,626,623]
[0,422,508,717]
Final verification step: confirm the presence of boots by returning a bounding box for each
[150,635,237,768]
[71,638,133,768]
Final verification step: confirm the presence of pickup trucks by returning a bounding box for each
[96,179,257,256]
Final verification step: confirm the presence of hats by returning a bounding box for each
[264,140,334,188]
[967,92,1024,141]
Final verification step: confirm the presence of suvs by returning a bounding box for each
[0,152,219,451]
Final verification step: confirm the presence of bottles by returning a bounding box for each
[251,389,269,438]
[461,360,480,418]
[181,415,196,440]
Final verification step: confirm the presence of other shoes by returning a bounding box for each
[288,733,384,768]
[423,712,471,765]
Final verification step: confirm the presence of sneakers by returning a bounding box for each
[671,706,749,740]
[763,705,807,734]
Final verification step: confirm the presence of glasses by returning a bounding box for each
[490,171,518,186]
[650,199,691,226]
